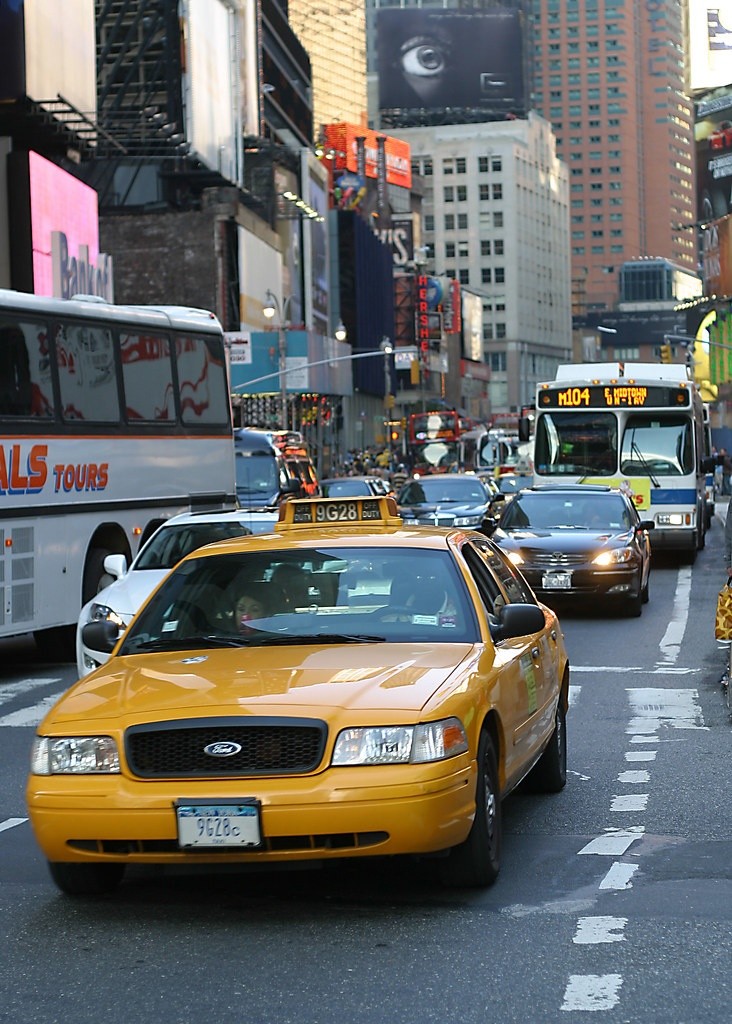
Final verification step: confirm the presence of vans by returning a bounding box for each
[233,427,319,506]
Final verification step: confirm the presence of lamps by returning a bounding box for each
[27,100,197,164]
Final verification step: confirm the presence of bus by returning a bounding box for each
[0,288,238,652]
[410,405,478,474]
[532,361,724,569]
[477,428,521,473]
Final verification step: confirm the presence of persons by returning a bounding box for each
[722,497,732,576]
[381,558,458,623]
[333,445,409,489]
[425,453,459,475]
[233,590,274,632]
[712,446,732,496]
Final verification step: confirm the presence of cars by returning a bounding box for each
[318,473,532,538]
[76,506,281,679]
[24,497,571,893]
[489,483,655,618]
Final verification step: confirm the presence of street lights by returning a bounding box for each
[328,317,348,471]
[379,333,395,447]
[262,287,302,430]
